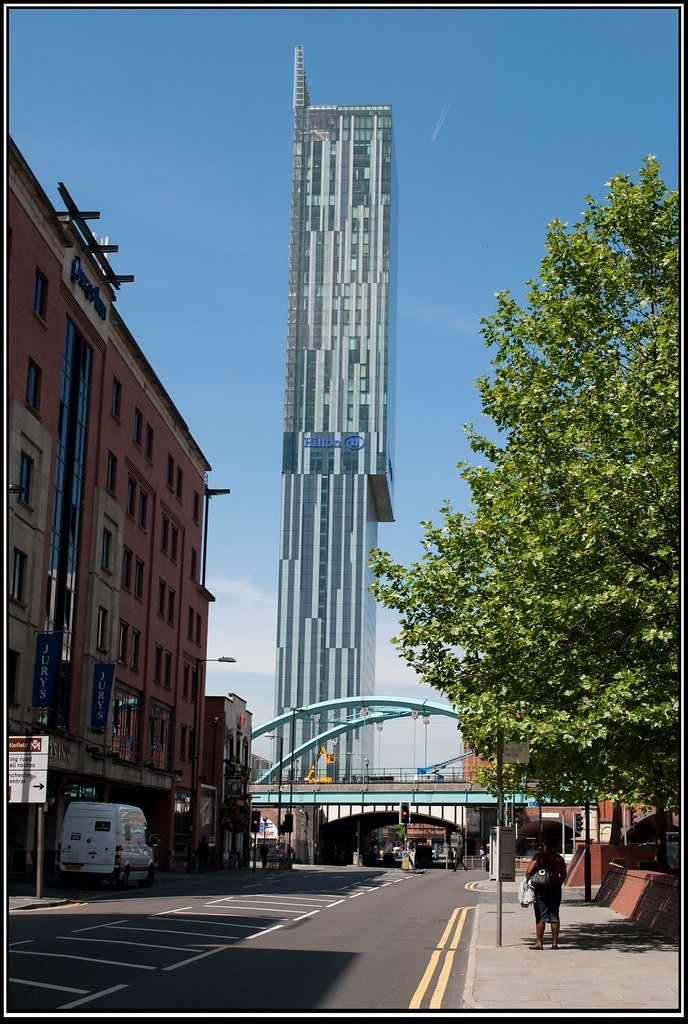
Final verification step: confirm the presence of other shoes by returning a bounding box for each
[551,944,558,949]
[529,945,544,950]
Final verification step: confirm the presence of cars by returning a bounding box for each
[383,853,396,862]
[250,842,295,863]
[638,831,679,854]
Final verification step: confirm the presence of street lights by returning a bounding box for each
[186,658,236,874]
[347,752,355,784]
[264,734,283,844]
[288,708,305,869]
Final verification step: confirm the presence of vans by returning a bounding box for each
[54,802,158,891]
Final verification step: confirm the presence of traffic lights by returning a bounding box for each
[575,814,583,832]
[627,807,637,827]
[511,804,520,826]
[250,811,261,833]
[399,802,410,825]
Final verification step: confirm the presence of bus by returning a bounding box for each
[364,776,394,784]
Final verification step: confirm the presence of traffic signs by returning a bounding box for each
[9,735,48,803]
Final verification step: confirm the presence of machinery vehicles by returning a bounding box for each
[304,746,334,784]
[415,750,476,783]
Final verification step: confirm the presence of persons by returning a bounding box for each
[453,841,468,872]
[523,842,567,950]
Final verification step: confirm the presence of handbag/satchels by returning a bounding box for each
[530,851,556,889]
